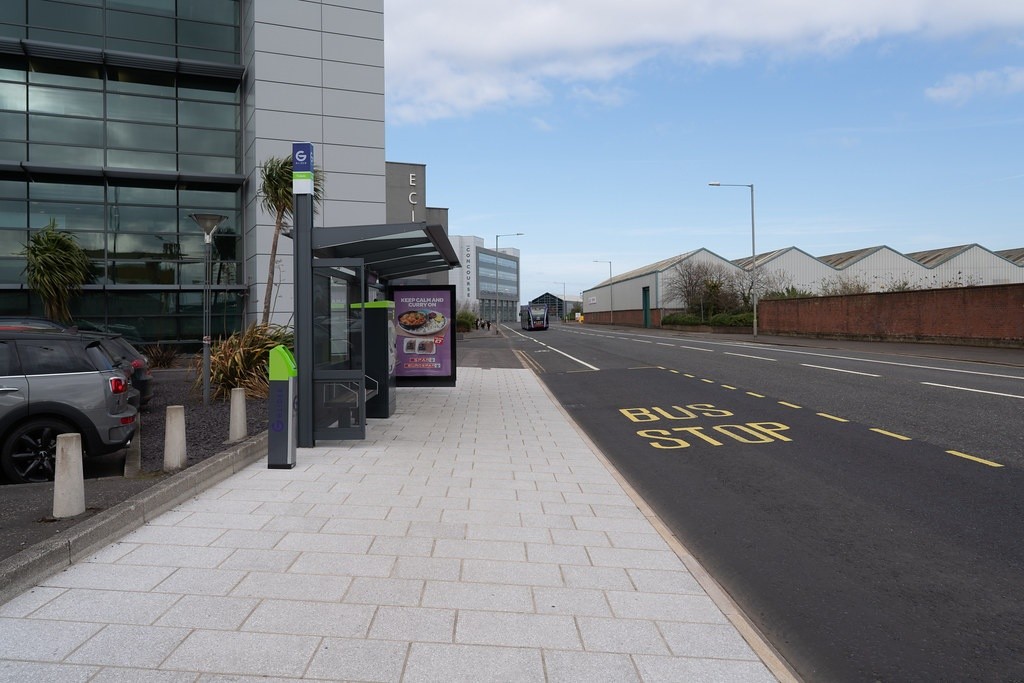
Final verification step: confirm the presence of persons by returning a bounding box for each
[487,319,492,331]
[476,318,485,330]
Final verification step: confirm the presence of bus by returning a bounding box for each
[519,303,550,331]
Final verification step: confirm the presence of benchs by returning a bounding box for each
[323,374,379,429]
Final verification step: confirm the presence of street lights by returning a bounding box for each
[553,282,566,323]
[495,232,524,335]
[707,182,758,337]
[593,261,613,326]
[188,211,230,406]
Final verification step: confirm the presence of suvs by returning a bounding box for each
[0,315,157,412]
[0,325,142,485]
